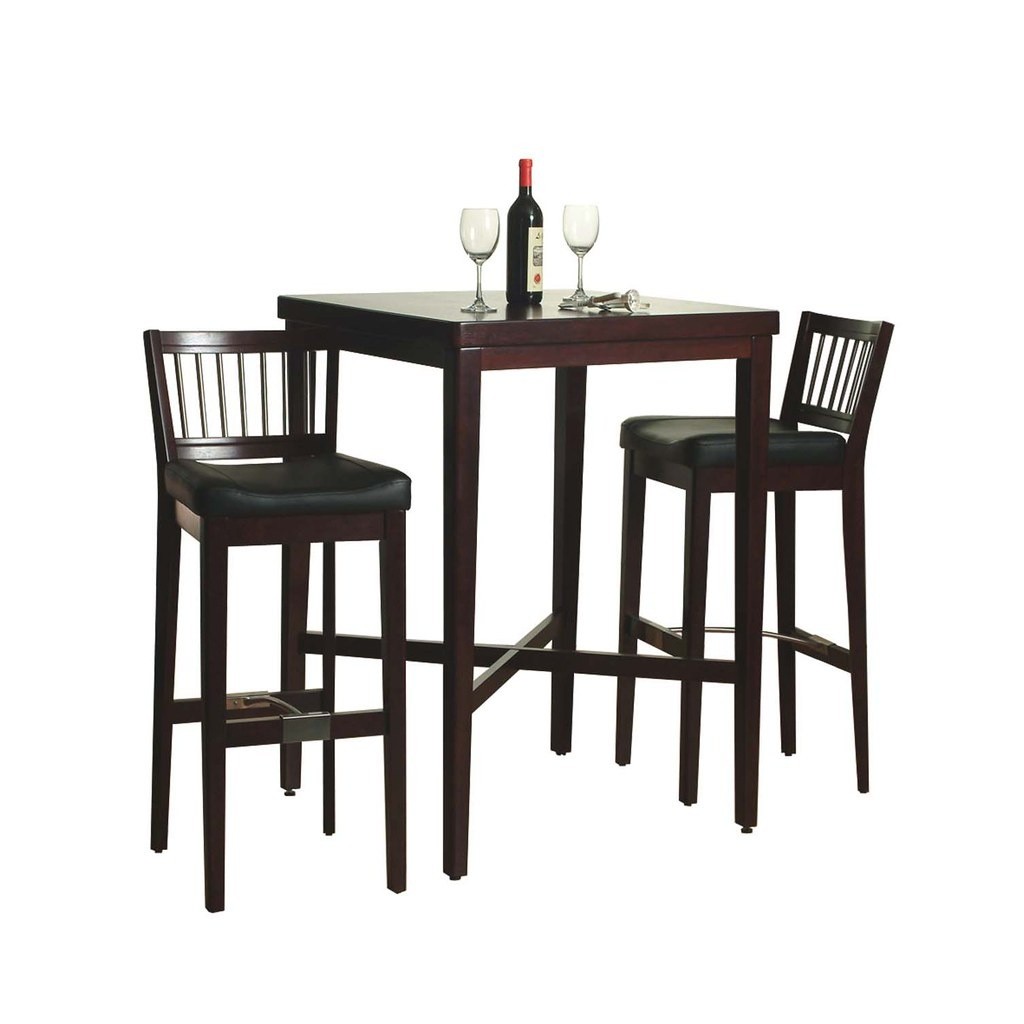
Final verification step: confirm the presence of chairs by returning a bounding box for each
[143,321,408,913]
[614,310,893,832]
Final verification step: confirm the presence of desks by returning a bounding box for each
[279,292,781,880]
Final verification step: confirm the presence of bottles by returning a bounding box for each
[506,158,544,304]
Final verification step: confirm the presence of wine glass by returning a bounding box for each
[562,204,599,301]
[460,208,500,314]
